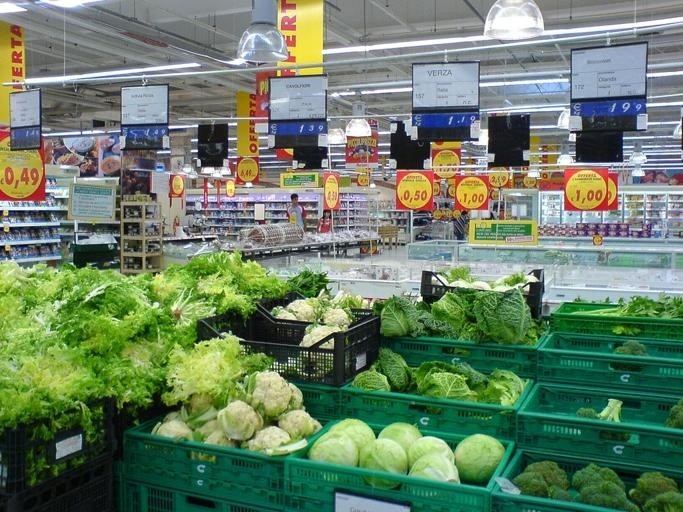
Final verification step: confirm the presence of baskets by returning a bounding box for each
[340,379,534,440]
[389,330,545,378]
[0,455,113,512]
[489,449,682,512]
[197,310,379,387]
[420,269,546,318]
[284,419,514,512]
[287,381,340,420]
[515,382,682,472]
[536,332,682,391]
[0,397,116,507]
[550,302,682,341]
[254,291,372,328]
[121,479,276,512]
[105,390,183,460]
[121,413,285,512]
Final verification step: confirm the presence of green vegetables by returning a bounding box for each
[566,294,683,336]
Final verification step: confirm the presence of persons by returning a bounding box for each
[316,210,330,234]
[640,170,683,186]
[431,201,453,222]
[453,210,468,240]
[285,194,308,231]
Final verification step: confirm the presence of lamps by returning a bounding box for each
[326,107,347,144]
[347,98,371,137]
[556,108,572,128]
[631,163,645,177]
[672,106,683,139]
[483,0,545,41]
[628,140,647,163]
[237,0,291,62]
[219,158,232,175]
[472,112,489,146]
[556,140,574,165]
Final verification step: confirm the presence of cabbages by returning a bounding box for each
[354,345,525,408]
[378,288,540,346]
[308,416,502,489]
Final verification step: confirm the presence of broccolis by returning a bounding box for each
[269,289,369,324]
[512,460,683,512]
[576,397,632,440]
[662,396,683,446]
[150,371,318,461]
[295,325,353,379]
[611,340,649,371]
